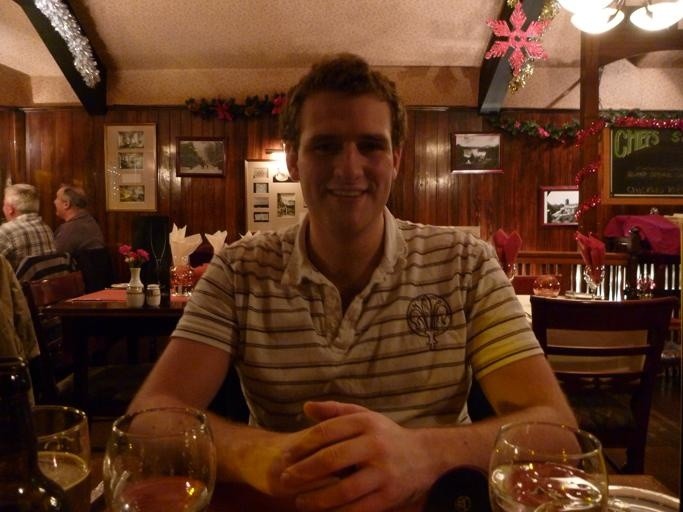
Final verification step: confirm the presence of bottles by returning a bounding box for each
[627,229,646,290]
[169,255,196,297]
[0,361,65,511]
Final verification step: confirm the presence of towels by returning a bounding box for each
[492,229,522,262]
[204,229,231,250]
[574,232,606,282]
[166,223,202,261]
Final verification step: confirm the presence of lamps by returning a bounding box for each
[558,1,681,34]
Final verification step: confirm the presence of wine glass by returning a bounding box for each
[584,264,605,300]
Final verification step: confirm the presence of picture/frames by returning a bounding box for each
[242,158,308,235]
[173,135,227,179]
[450,131,504,174]
[537,186,579,231]
[600,124,683,207]
[100,121,157,214]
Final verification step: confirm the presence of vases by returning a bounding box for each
[126,268,147,307]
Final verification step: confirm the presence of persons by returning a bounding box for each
[0,250,42,411]
[0,181,57,324]
[124,46,583,512]
[53,183,106,273]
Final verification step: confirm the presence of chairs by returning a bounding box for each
[528,293,677,474]
[19,270,158,405]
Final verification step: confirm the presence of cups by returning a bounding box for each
[35,403,90,510]
[499,263,516,282]
[146,285,161,295]
[100,406,217,512]
[488,421,609,512]
[639,274,652,297]
[146,295,159,307]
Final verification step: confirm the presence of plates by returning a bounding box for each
[602,483,679,512]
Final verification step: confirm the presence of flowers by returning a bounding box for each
[120,245,149,269]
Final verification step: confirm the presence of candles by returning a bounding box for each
[573,263,683,301]
